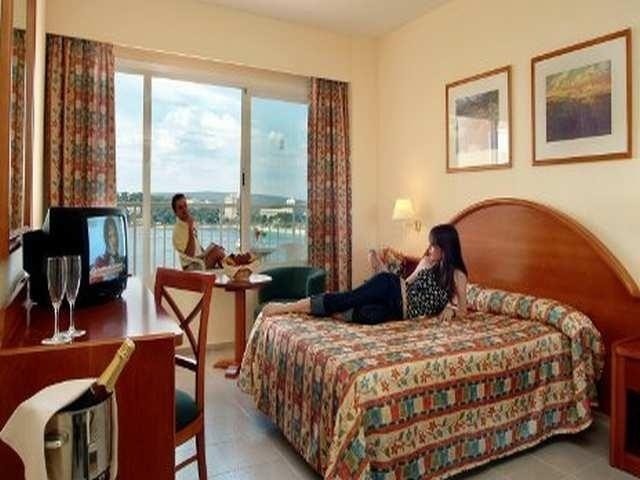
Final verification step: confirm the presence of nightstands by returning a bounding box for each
[608,333,640,479]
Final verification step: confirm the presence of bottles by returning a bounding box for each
[79,338,136,408]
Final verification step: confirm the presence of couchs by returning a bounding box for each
[253,267,327,321]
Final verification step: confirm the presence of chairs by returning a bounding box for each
[155,268,217,480]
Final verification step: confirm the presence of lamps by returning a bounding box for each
[392,197,422,231]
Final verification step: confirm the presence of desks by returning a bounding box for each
[210,272,272,378]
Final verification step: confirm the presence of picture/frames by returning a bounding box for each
[0,0,36,263]
[531,27,634,166]
[445,66,512,172]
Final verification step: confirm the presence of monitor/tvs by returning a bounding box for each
[22,205,129,310]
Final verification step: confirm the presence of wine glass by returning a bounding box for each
[61,254,86,338]
[41,257,72,345]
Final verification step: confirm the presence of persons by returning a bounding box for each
[171,193,227,271]
[261,223,469,324]
[94,218,120,280]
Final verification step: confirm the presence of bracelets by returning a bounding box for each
[452,309,456,319]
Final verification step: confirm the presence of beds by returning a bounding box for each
[238,199,640,480]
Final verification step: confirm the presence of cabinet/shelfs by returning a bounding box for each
[0,276,182,480]
